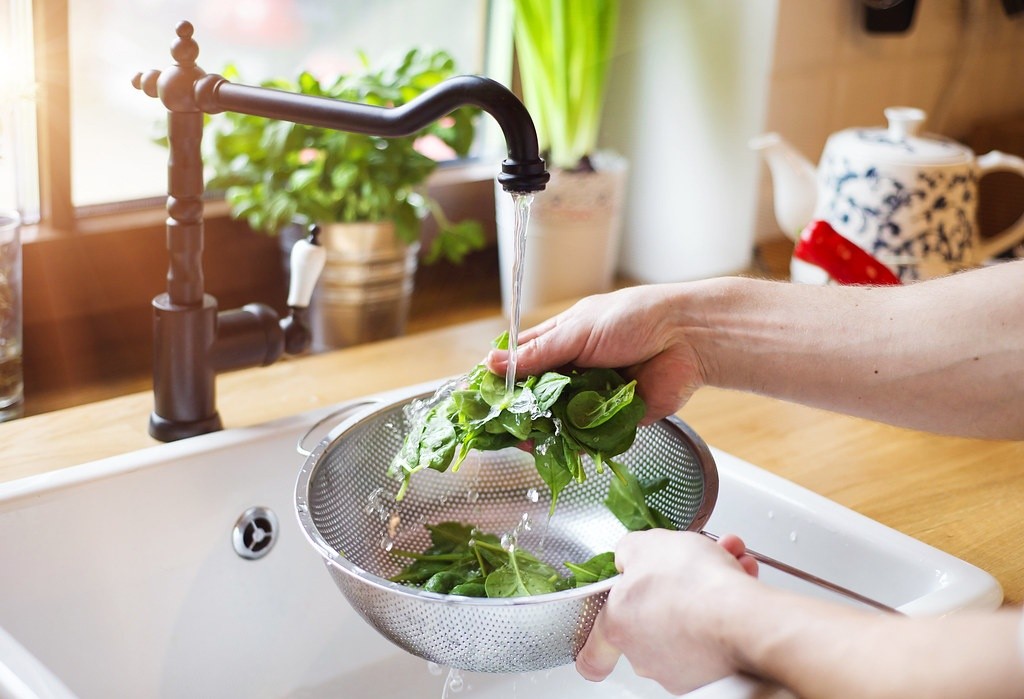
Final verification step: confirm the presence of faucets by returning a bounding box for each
[130,19,551,445]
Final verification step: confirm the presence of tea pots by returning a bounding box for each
[752,105,1023,287]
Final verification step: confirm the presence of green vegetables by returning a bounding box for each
[388,329,677,600]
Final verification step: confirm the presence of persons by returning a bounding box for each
[487,255,1024,699]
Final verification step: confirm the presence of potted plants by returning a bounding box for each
[491,0,629,318]
[152,44,488,363]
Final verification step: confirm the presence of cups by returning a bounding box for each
[0,210,26,422]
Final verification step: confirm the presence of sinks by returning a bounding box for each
[0,374,1006,699]
